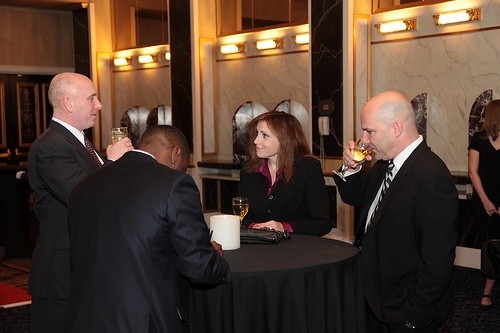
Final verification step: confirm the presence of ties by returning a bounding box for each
[83,134,102,169]
[367,159,394,230]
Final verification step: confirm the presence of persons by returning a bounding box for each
[333,89,459,333]
[67,125,229,333]
[26,71,135,333]
[237,111,333,237]
[465,99,500,314]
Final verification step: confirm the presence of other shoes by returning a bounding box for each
[480,295,492,310]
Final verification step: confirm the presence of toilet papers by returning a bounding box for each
[210,215,240,250]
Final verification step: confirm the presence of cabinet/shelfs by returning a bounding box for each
[197,160,355,244]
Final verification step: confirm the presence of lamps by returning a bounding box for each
[255,38,283,49]
[293,33,309,45]
[374,18,416,35]
[431,8,481,27]
[220,44,245,54]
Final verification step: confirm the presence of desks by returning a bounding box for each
[181,235,367,332]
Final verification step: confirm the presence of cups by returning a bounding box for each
[210,215,241,251]
[232,197,250,226]
[112,127,128,144]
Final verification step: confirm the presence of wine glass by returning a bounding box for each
[332,138,373,183]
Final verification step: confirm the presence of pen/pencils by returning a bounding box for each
[210,230,213,241]
